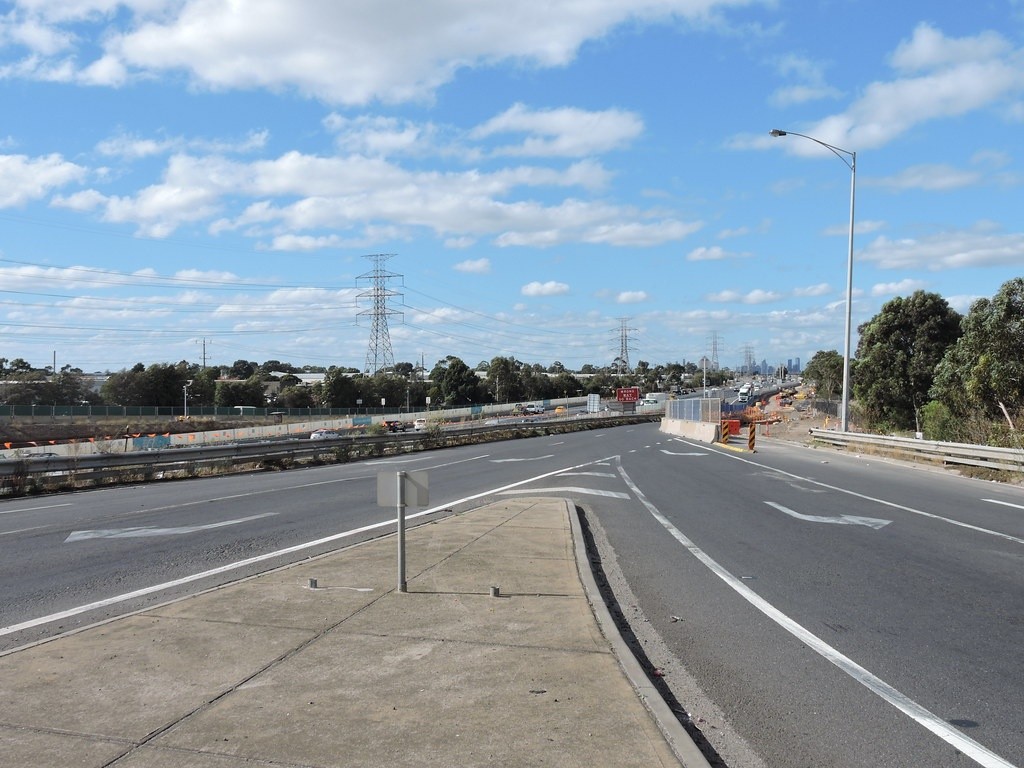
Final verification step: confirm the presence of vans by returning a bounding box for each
[414,418,434,431]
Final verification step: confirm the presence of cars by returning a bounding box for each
[310,429,340,439]
[554,406,568,416]
[727,376,797,393]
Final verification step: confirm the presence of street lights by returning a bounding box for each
[182,379,195,419]
[769,129,857,433]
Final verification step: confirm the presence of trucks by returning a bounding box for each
[640,384,686,407]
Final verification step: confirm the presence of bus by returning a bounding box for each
[738,384,752,402]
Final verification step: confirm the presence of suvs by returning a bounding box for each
[526,404,546,414]
[384,421,408,433]
